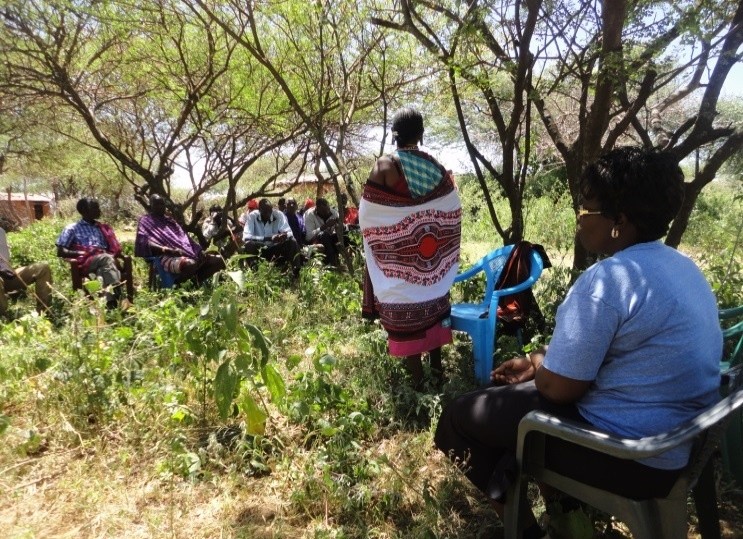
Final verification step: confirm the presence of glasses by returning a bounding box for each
[578,205,603,219]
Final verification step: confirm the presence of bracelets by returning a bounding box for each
[526,353,532,365]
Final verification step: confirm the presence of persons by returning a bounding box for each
[57,198,140,313]
[359,108,462,393]
[433,145,723,539]
[204,193,359,278]
[0,227,62,328]
[135,194,233,297]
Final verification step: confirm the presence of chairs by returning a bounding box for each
[448,245,545,384]
[503,305,743,539]
[67,252,174,306]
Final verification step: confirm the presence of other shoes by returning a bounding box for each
[480,522,544,539]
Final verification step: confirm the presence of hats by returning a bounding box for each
[304,200,314,208]
[248,200,258,209]
